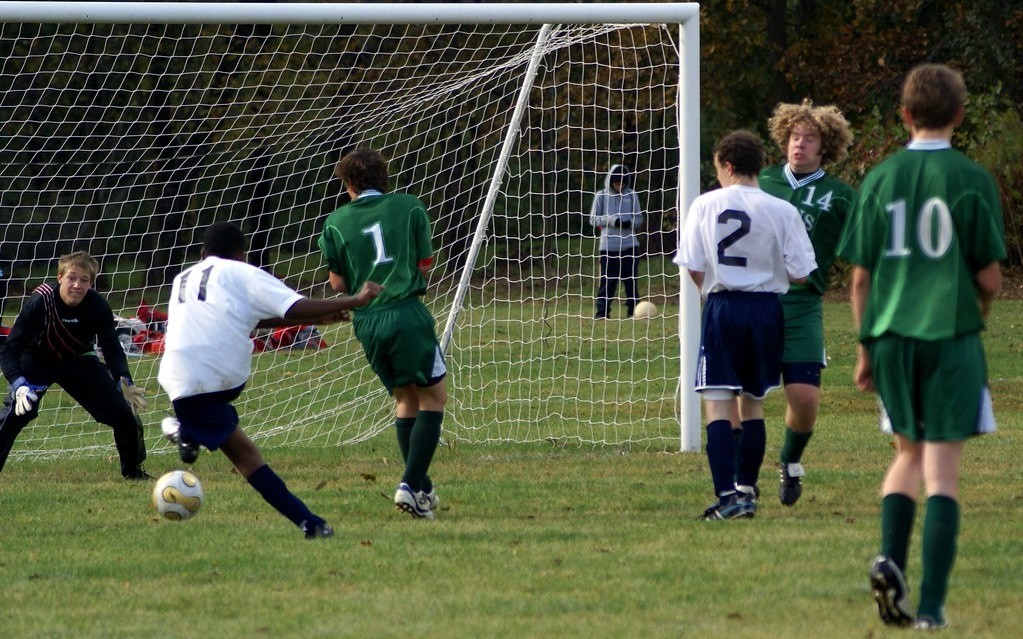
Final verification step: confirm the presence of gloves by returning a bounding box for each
[116,375,147,417]
[616,218,631,229]
[10,375,48,417]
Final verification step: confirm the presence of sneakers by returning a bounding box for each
[695,490,747,524]
[778,461,805,505]
[735,484,761,517]
[914,614,948,630]
[870,556,913,627]
[161,416,200,463]
[394,480,439,521]
[305,523,335,539]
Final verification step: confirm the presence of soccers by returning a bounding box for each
[634,300,658,320]
[152,468,204,522]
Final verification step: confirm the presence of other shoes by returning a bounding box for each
[627,311,633,318]
[597,311,609,320]
[121,464,158,481]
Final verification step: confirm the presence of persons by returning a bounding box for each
[836,63,1006,630]
[674,129,817,520]
[757,102,857,507]
[0,251,157,481]
[589,164,643,319]
[317,148,448,521]
[158,222,383,540]
